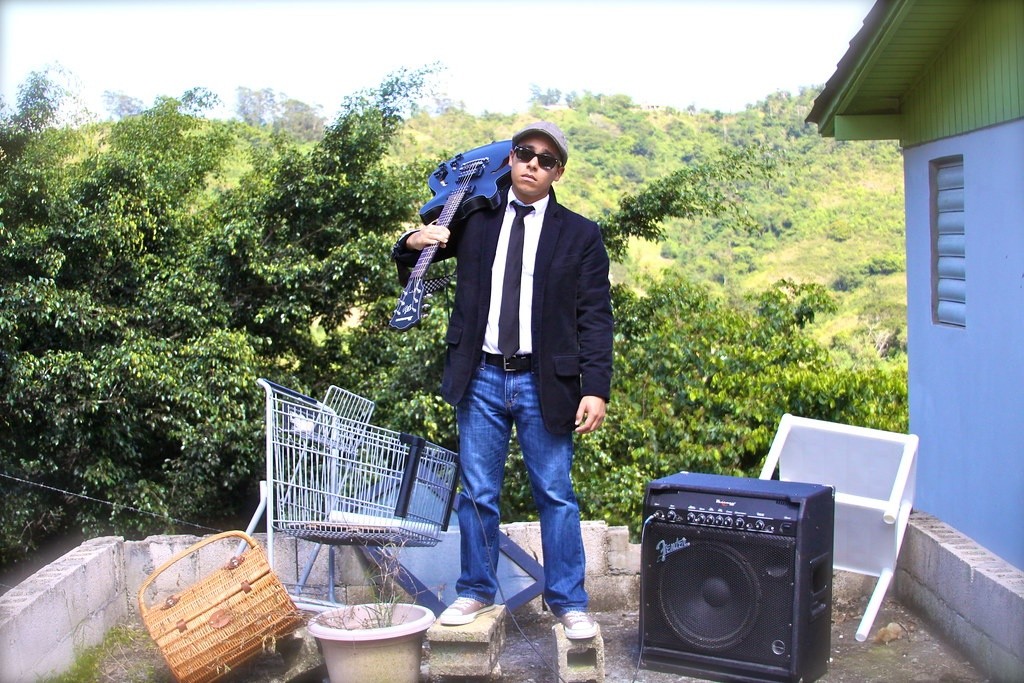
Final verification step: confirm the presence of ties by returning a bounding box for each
[498,201,535,360]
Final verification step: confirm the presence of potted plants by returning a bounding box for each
[303,531,436,683]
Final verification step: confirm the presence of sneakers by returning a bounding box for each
[440,597,495,625]
[560,611,598,640]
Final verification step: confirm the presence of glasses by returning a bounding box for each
[514,146,562,170]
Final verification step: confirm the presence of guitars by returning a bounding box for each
[388,140,515,334]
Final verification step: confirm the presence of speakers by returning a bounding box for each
[636,471,836,683]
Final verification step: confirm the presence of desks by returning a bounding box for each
[757,415,919,642]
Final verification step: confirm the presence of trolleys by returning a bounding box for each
[256,377,458,615]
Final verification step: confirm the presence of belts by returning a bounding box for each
[485,353,532,371]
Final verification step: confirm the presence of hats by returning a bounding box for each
[512,121,568,166]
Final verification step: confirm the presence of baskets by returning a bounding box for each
[138,530,305,683]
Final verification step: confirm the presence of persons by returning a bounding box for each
[392,119,614,639]
